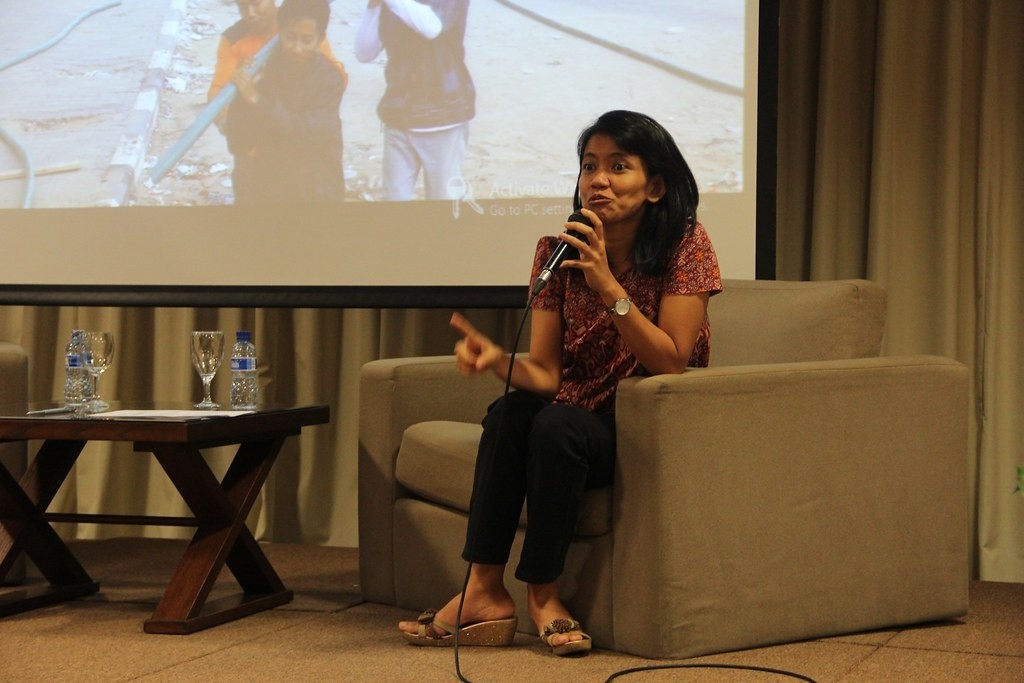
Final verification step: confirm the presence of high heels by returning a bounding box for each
[402,608,518,646]
[538,617,592,655]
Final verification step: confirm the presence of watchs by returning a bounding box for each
[608,296,632,317]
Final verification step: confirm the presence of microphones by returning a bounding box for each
[532,209,594,295]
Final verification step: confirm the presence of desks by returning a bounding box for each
[0,402,331,636]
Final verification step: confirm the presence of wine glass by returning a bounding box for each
[82,332,115,414]
[190,330,227,409]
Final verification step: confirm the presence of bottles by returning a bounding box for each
[229,331,259,412]
[64,329,93,405]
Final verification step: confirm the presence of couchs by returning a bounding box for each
[358,279,971,659]
[0,340,28,588]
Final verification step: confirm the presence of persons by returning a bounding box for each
[398,111,723,654]
[355,0,475,203]
[227,0,345,199]
[207,0,348,151]
